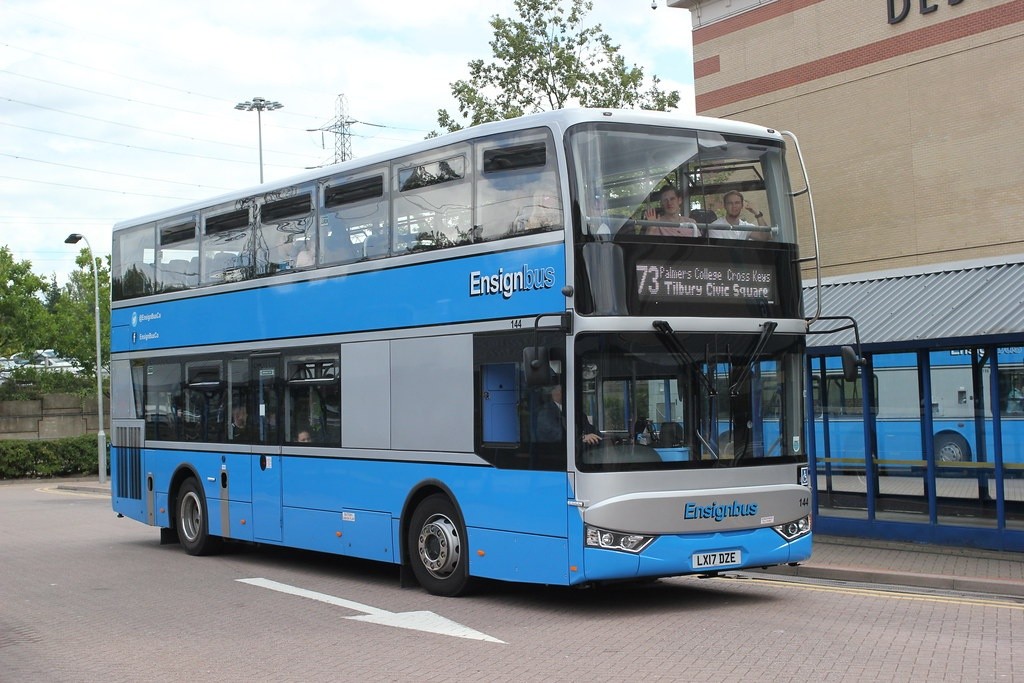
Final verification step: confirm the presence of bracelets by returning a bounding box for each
[755,211,763,218]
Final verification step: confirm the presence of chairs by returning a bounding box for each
[363,234,387,257]
[290,239,310,268]
[528,385,542,442]
[601,214,636,234]
[689,208,717,236]
[122,262,148,296]
[639,208,664,235]
[165,259,190,284]
[142,262,169,294]
[209,253,237,282]
[186,256,213,285]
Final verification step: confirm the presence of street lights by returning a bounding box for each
[233,97,285,185]
[63,233,108,484]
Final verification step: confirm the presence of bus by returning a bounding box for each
[649,347,1024,478]
[108,109,869,600]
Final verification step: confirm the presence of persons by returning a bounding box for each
[294,430,311,443]
[643,185,702,238]
[706,190,773,240]
[535,383,603,445]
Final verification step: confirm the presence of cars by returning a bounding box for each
[0,347,107,387]
